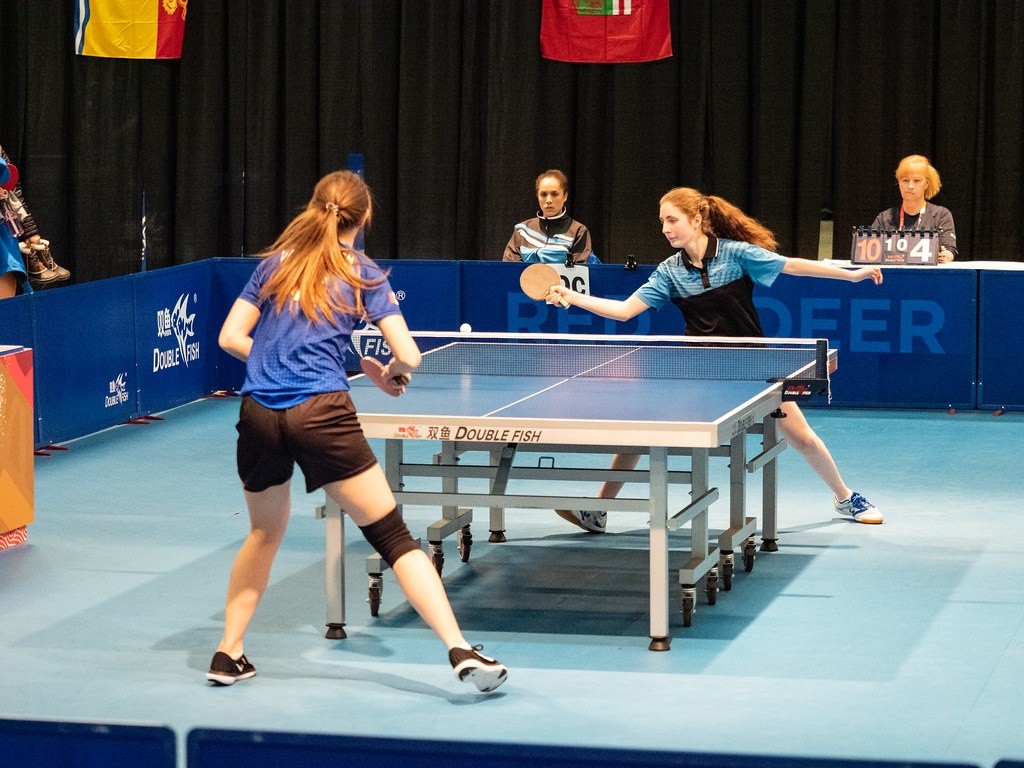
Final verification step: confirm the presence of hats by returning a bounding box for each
[0,157,19,190]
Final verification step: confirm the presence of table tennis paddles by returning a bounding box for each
[360,356,411,397]
[519,263,571,310]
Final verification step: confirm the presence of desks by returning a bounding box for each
[315,331,837,651]
[820,260,1024,272]
[0,345,35,553]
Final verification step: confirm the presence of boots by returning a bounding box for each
[26,239,70,281]
[18,242,59,283]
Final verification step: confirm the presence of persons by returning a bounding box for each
[0,145,40,301]
[545,188,883,533]
[502,169,591,264]
[203,168,509,694]
[870,155,959,263]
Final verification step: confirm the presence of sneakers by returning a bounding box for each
[555,510,607,533]
[205,651,256,685]
[833,491,883,524]
[449,644,508,692]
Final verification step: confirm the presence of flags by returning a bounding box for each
[539,0,674,64]
[72,0,187,58]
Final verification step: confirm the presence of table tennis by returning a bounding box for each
[460,323,472,333]
